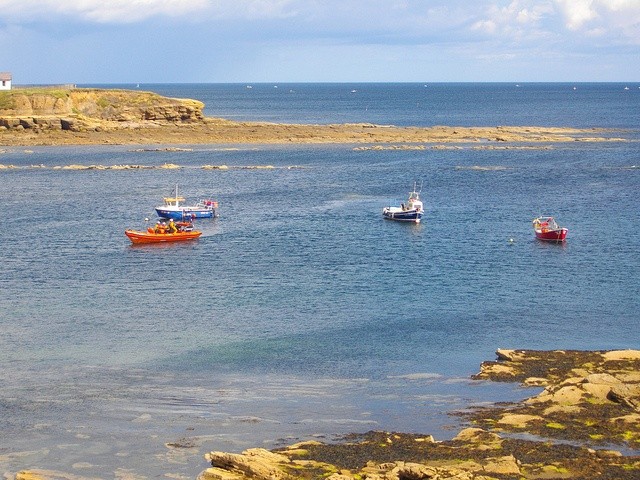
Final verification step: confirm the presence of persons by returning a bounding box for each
[153,218,177,238]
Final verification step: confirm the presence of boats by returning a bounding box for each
[533,216,568,242]
[155,183,218,221]
[124,207,202,245]
[382,179,423,225]
